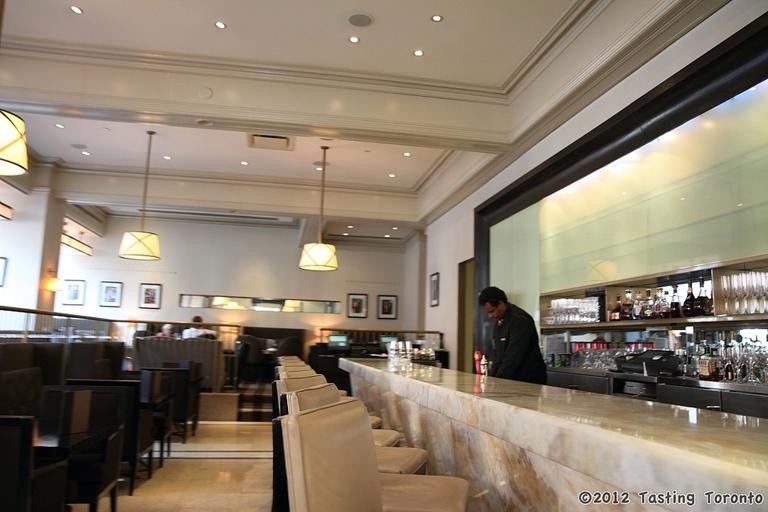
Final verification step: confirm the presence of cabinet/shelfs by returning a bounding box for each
[605,263,715,327]
[710,254,768,322]
[656,383,768,420]
[547,371,608,394]
[539,282,605,328]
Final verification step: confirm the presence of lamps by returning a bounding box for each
[119,131,161,260]
[298,146,338,272]
[0,108,29,176]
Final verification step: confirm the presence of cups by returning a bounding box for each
[386,340,413,367]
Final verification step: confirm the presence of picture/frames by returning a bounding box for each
[347,294,368,318]
[138,283,162,309]
[430,273,439,307]
[378,295,397,319]
[99,281,123,308]
[61,279,87,305]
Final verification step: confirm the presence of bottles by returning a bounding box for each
[686,341,767,385]
[611,278,714,320]
[568,340,653,372]
[473,350,488,376]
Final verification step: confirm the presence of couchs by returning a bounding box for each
[133,322,220,339]
[320,328,444,350]
[133,339,225,392]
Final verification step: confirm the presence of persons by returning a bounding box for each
[182,316,217,339]
[156,324,181,340]
[478,287,547,385]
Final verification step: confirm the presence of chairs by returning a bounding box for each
[272,355,470,512]
[0,360,203,512]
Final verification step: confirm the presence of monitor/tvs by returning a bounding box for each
[329,335,348,342]
[380,336,400,343]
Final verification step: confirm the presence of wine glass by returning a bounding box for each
[543,296,602,325]
[719,270,767,315]
